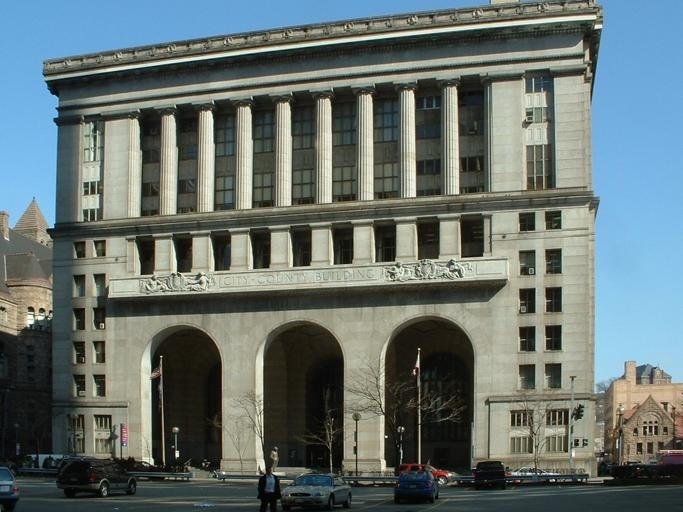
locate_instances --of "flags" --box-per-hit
[411,353,418,377]
[149,366,160,381]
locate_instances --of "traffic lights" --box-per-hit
[576,404,584,419]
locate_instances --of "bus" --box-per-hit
[21,453,63,470]
[657,449,682,478]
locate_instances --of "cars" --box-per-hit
[0,466,19,512]
[508,466,561,484]
[393,471,439,504]
[279,473,352,511]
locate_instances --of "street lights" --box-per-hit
[351,413,361,485]
[615,405,624,465]
[171,426,179,480]
[396,425,405,464]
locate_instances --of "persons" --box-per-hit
[270,446,279,472]
[145,275,168,294]
[449,259,464,278]
[387,262,404,280]
[188,272,210,291]
[256,466,281,512]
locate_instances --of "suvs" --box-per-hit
[623,460,641,465]
[394,463,455,485]
[55,460,137,498]
[475,460,506,489]
[56,456,93,475]
